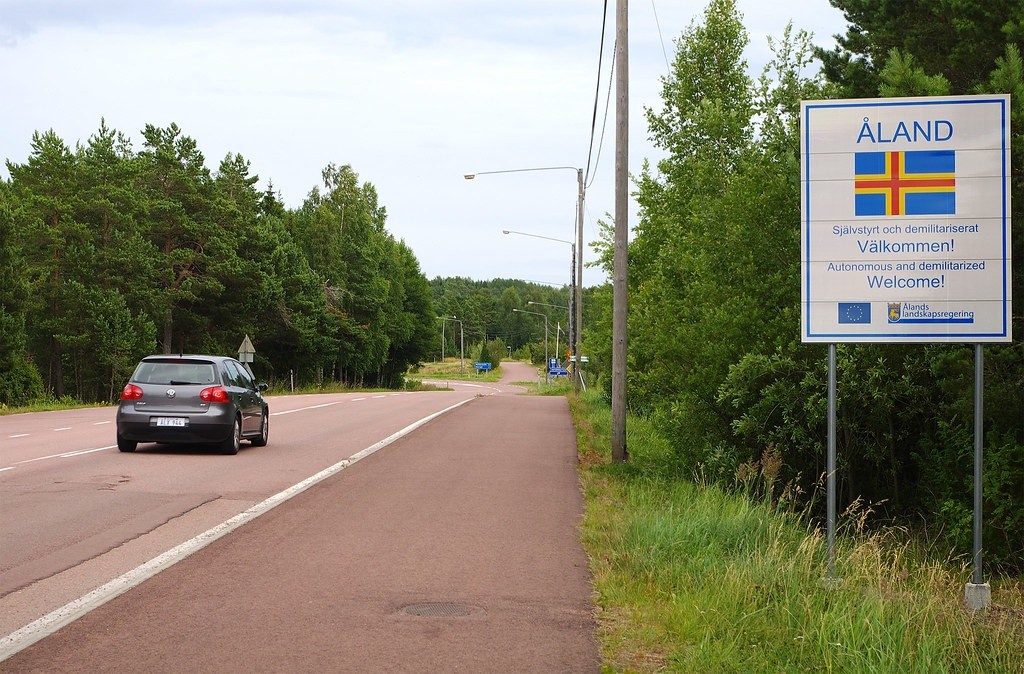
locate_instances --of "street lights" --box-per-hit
[502,230,575,384]
[529,301,572,359]
[513,309,548,385]
[464,167,581,397]
[435,317,463,378]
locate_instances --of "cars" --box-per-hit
[116,355,270,455]
